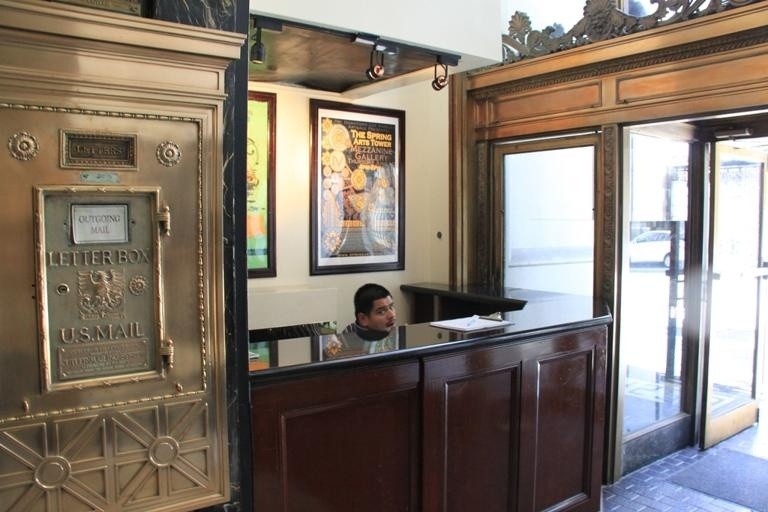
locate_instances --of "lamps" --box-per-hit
[250,28,267,65]
[432,64,450,90]
[366,47,385,81]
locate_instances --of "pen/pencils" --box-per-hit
[467,314,480,328]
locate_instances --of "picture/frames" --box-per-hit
[246,88,278,280]
[309,98,405,276]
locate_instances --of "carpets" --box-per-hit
[668,448,767,510]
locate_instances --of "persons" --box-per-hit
[340,284,396,334]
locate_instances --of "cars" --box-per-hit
[627,230,686,269]
